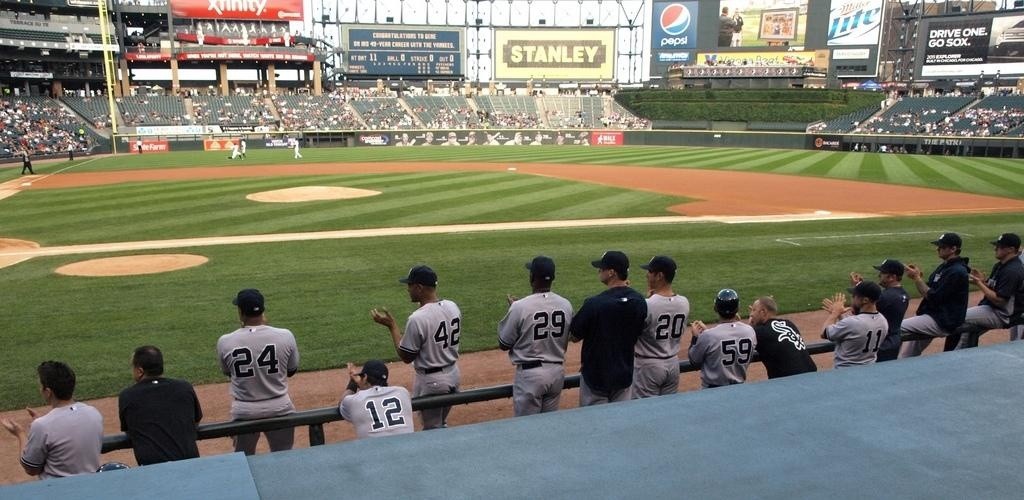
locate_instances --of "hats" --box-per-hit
[525,256,555,280]
[930,233,962,248]
[639,255,677,272]
[399,265,438,286]
[232,288,265,314]
[353,360,388,382]
[872,259,904,276]
[846,280,881,299]
[591,250,629,271]
[990,233,1021,247]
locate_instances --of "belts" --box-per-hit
[522,361,542,369]
[425,367,442,375]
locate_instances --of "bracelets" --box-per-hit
[347,378,357,392]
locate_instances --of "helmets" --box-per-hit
[715,288,739,314]
[96,461,130,473]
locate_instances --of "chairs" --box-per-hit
[817,94,1024,138]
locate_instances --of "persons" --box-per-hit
[225,143,243,160]
[962,233,1024,348]
[1,360,105,482]
[632,256,690,400]
[196,20,287,36]
[555,132,565,145]
[718,7,743,47]
[748,295,817,379]
[688,288,758,389]
[118,346,203,467]
[370,263,463,430]
[467,132,479,145]
[850,259,910,362]
[484,134,500,145]
[240,139,248,159]
[216,288,299,455]
[396,133,414,147]
[819,281,889,369]
[503,132,524,145]
[899,232,971,359]
[62,87,102,98]
[578,132,589,138]
[569,251,648,407]
[422,132,434,146]
[529,132,543,145]
[294,138,302,159]
[497,254,574,418]
[853,143,961,156]
[440,132,460,146]
[337,361,416,439]
[0,98,85,175]
[926,106,1024,138]
[137,138,142,152]
